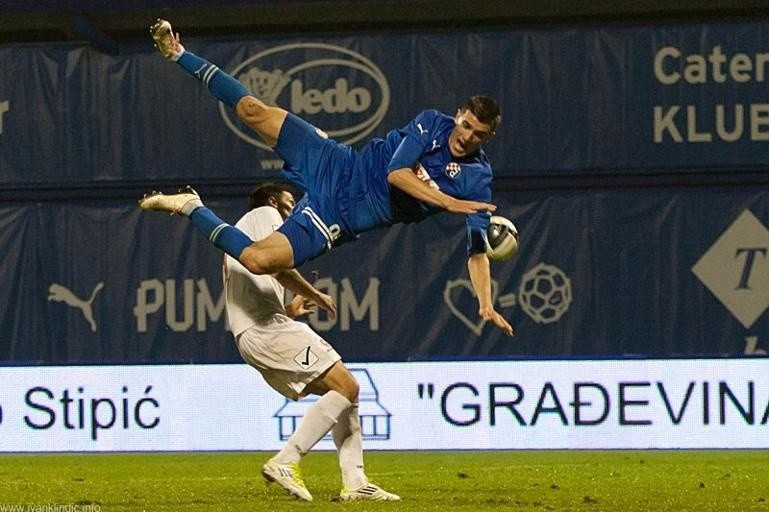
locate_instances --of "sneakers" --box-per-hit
[150,17,181,59]
[261,457,313,502]
[338,482,400,505]
[138,184,201,217]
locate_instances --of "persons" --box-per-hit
[137,17,514,339]
[220,180,403,503]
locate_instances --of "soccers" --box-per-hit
[489,216,518,260]
